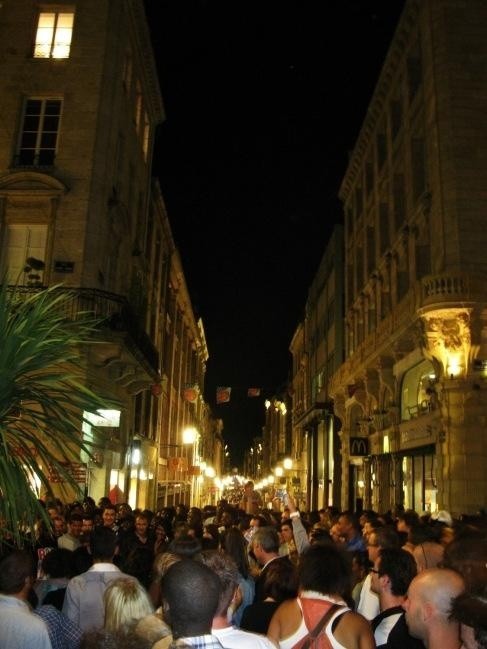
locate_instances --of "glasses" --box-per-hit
[362,541,375,547]
[368,566,378,575]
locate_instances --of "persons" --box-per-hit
[1,481,487,649]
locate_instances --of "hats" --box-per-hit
[430,509,451,525]
